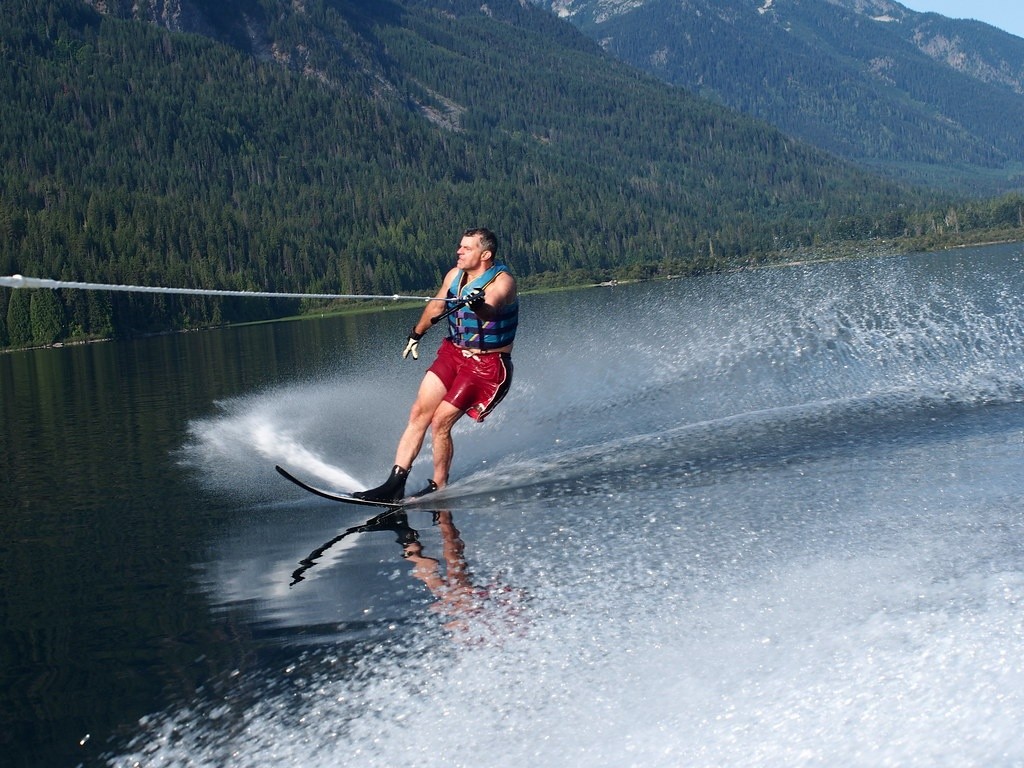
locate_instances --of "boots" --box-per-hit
[405,474,449,503]
[358,510,419,549]
[353,465,411,503]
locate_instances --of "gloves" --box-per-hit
[402,326,427,360]
[462,286,485,311]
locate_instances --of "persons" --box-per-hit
[353,227,518,503]
[364,510,536,648]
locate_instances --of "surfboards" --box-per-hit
[274,464,410,507]
